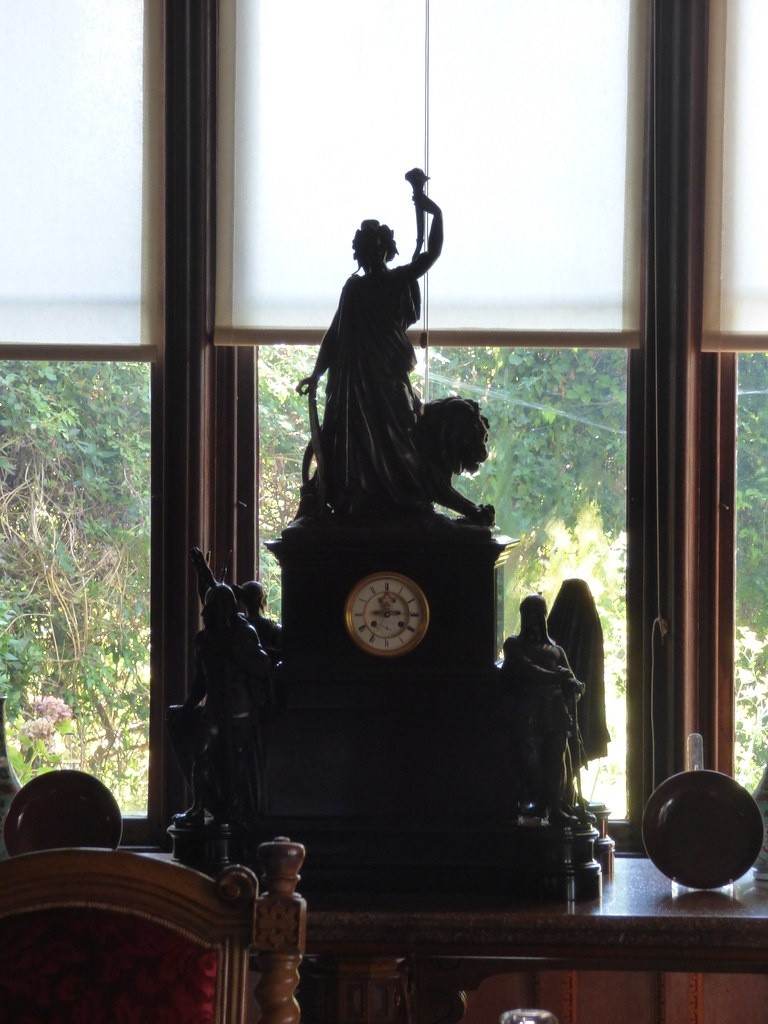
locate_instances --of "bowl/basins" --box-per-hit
[4,770,123,858]
[641,770,764,889]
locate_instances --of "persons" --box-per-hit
[294,193,443,490]
[171,584,277,826]
[190,548,292,666]
[503,596,586,815]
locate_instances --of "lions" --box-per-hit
[294,395,500,523]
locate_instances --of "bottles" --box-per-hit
[756,769,768,881]
[0,699,18,858]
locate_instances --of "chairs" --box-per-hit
[0,846,259,1024]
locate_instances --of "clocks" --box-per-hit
[345,570,430,657]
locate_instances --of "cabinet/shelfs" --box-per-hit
[296,940,768,1024]
